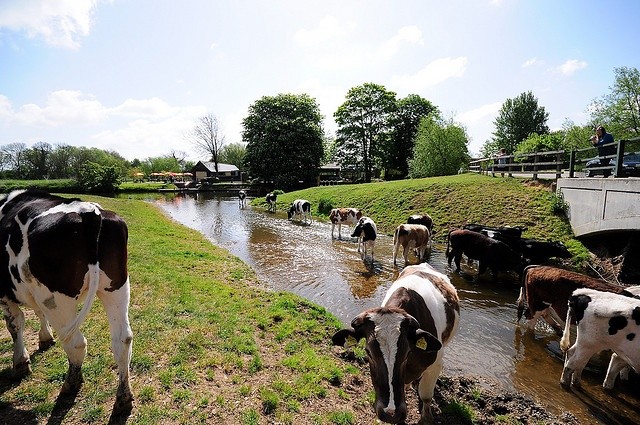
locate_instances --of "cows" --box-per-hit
[238,190,246,206]
[0,189,135,413]
[559,288,640,390]
[333,261,460,424]
[287,199,312,224]
[351,216,377,261]
[519,238,572,259]
[266,193,277,212]
[445,228,527,279]
[393,223,431,264]
[516,264,640,339]
[461,223,528,263]
[329,208,362,239]
[408,214,433,254]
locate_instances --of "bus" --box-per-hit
[317,166,341,183]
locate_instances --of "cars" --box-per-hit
[586,153,640,177]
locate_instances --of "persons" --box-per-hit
[591,125,615,178]
[498,148,510,178]
